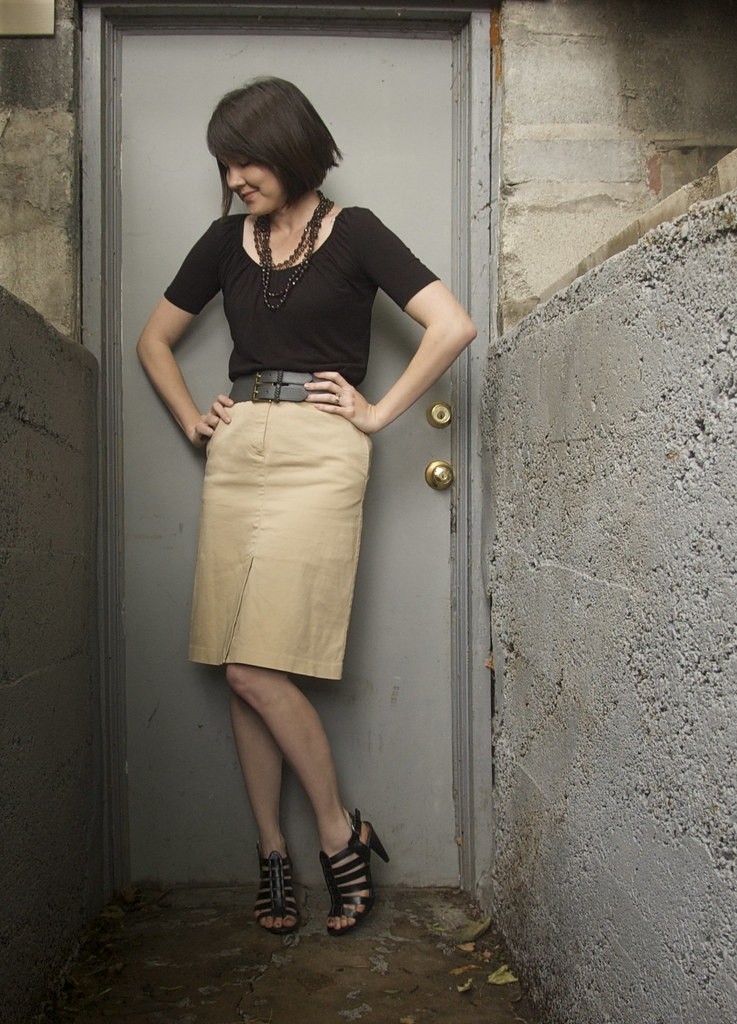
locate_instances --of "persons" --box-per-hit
[135,78,476,935]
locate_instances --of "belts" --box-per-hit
[229,370,335,403]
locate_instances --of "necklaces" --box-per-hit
[253,188,336,311]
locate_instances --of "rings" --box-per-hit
[334,393,340,406]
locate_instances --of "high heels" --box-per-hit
[253,839,300,935]
[319,808,390,937]
